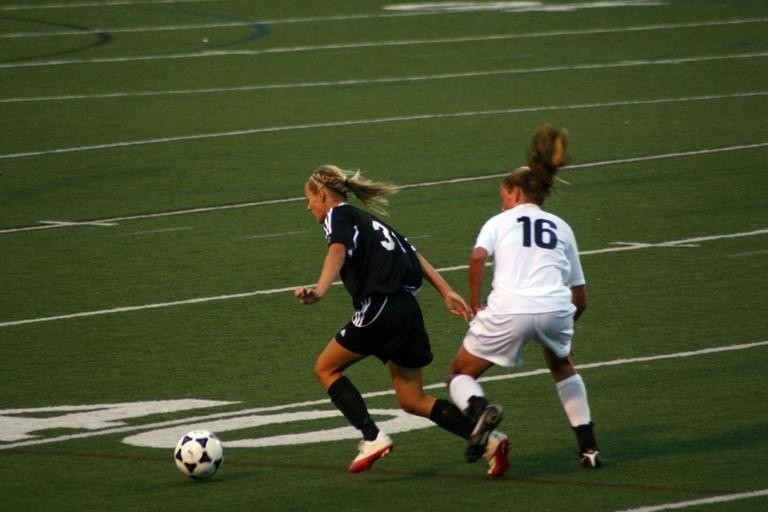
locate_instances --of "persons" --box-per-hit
[294,162,511,482]
[443,123,603,474]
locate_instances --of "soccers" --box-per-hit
[174,429,224,479]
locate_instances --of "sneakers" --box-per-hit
[580,449,603,470]
[483,429,511,480]
[348,429,394,474]
[464,395,503,464]
